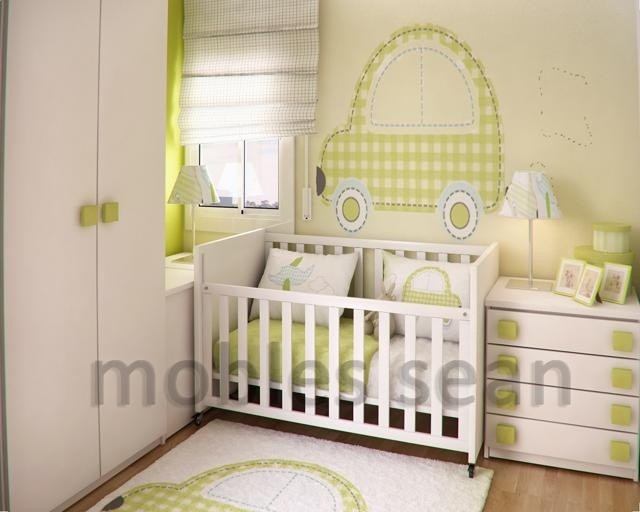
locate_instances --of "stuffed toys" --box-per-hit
[364,280,399,339]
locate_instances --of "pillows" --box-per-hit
[382,249,473,344]
[249,251,359,327]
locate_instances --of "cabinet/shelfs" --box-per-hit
[0,0,185,464]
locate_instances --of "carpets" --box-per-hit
[86,419,495,512]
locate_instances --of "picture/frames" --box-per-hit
[553,258,586,297]
[597,261,632,305]
[574,264,604,306]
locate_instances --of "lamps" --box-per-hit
[499,170,562,291]
[168,165,220,265]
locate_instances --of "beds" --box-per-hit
[194,221,498,478]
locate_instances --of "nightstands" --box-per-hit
[484,276,640,484]
